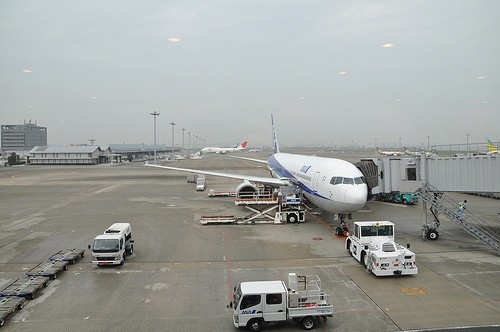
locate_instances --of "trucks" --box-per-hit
[230,272,333,332]
[187,174,206,191]
[88,222,135,266]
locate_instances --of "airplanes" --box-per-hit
[200,140,248,154]
[144,113,368,236]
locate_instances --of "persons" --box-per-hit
[342,225,348,238]
[456,200,468,221]
